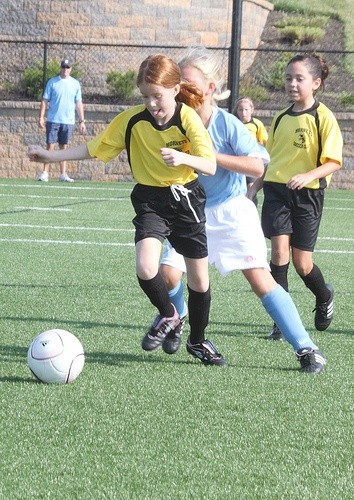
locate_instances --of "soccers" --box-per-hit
[27,329,85,383]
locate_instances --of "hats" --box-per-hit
[61,60,72,67]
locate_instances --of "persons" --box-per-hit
[230,96,268,207]
[25,54,225,366]
[37,58,86,182]
[246,51,344,341]
[158,47,328,376]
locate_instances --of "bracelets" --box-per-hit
[79,119,85,123]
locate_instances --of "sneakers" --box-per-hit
[142,302,180,351]
[38,172,48,181]
[312,284,334,330]
[59,174,73,182]
[186,336,225,367]
[162,318,184,354]
[295,347,326,373]
[270,324,285,342]
